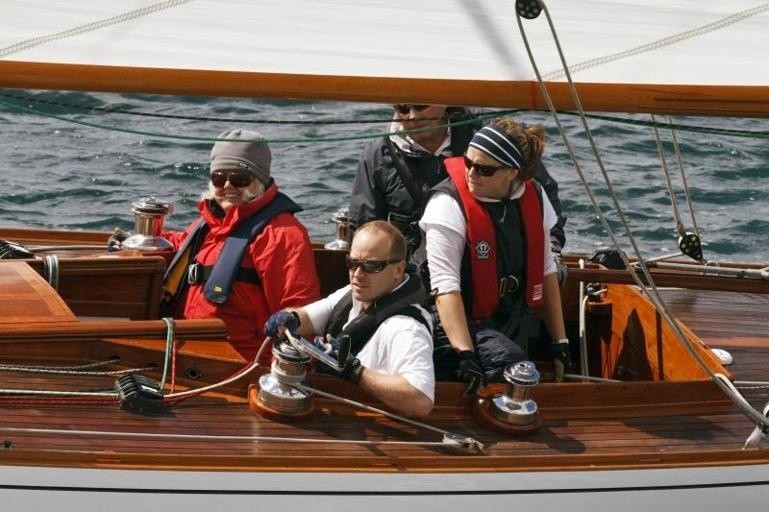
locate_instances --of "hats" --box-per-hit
[211,130,271,185]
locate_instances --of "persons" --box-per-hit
[335,103,569,288]
[107,128,322,374]
[262,220,436,419]
[418,116,572,400]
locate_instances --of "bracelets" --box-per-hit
[549,339,568,344]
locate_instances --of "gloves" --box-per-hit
[108,228,131,251]
[264,312,300,341]
[549,339,572,370]
[313,335,364,384]
[454,351,488,397]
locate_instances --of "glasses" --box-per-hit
[211,172,250,187]
[346,257,390,273]
[394,104,430,114]
[463,155,506,176]
